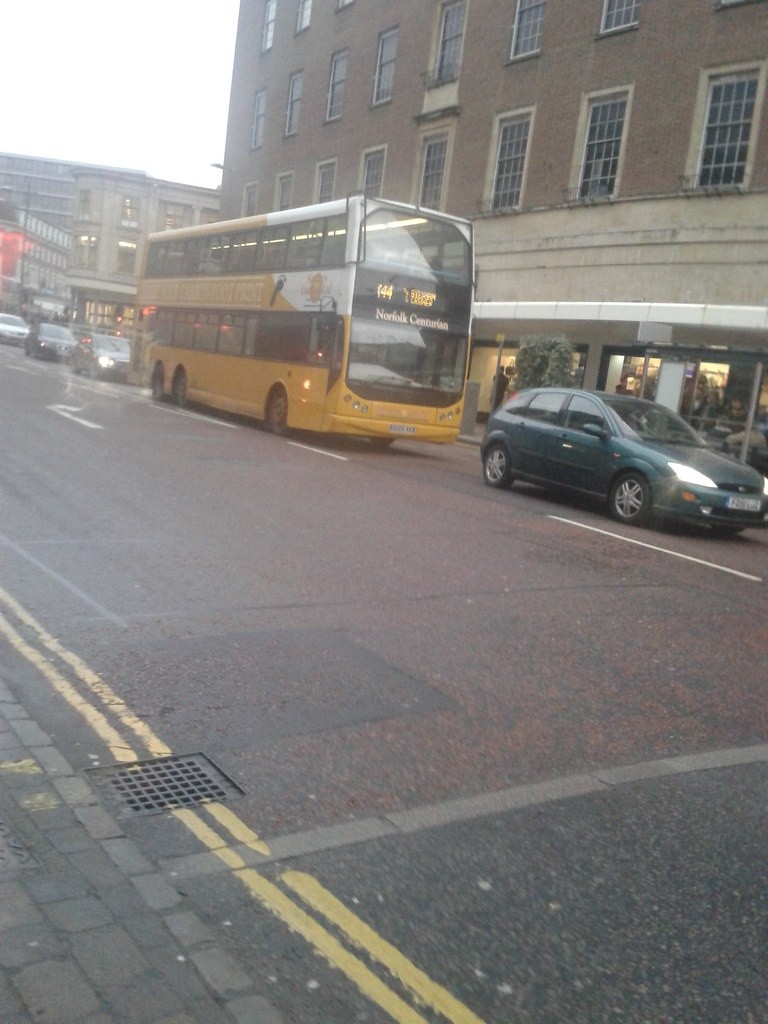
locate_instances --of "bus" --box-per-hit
[127,196,478,451]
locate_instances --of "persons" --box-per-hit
[616,385,655,400]
[490,366,509,410]
[350,342,376,364]
[41,311,70,322]
[685,382,768,475]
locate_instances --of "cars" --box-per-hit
[479,385,768,536]
[22,321,74,362]
[0,314,27,347]
[66,332,134,381]
